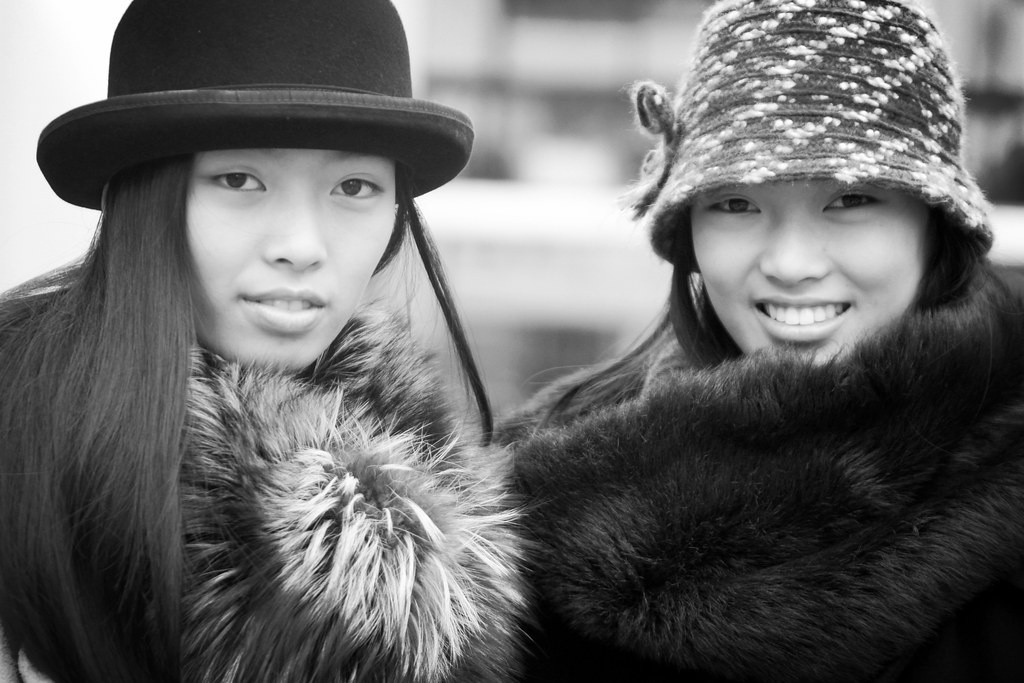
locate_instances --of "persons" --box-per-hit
[505,0,1024,683]
[0,0,535,683]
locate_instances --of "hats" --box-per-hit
[631,0,995,273]
[35,0,474,212]
